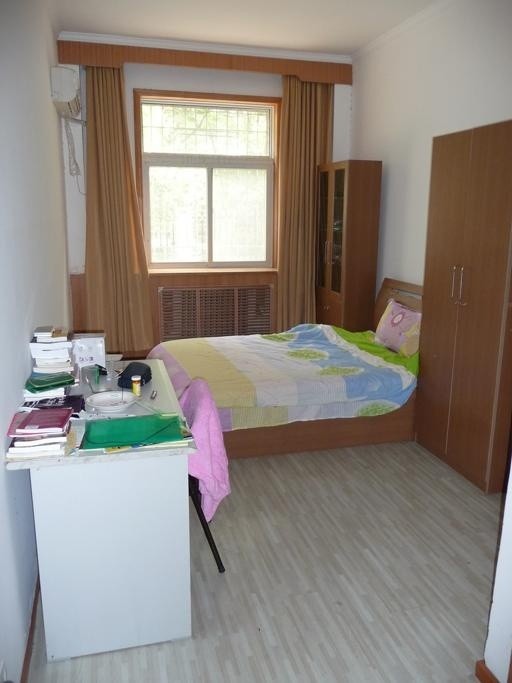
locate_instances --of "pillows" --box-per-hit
[375,300,422,353]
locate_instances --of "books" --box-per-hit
[6,327,79,461]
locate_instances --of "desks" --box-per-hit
[5,358,191,660]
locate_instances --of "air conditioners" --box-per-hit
[50,63,84,118]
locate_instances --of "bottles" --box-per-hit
[130,375,142,398]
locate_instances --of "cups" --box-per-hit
[81,366,100,399]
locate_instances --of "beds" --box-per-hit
[150,278,423,463]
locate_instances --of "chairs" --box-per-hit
[178,376,230,574]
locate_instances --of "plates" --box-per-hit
[87,391,138,412]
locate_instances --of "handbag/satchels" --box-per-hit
[117,361,152,388]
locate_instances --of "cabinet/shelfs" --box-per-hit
[316,159,381,332]
[413,118,512,495]
